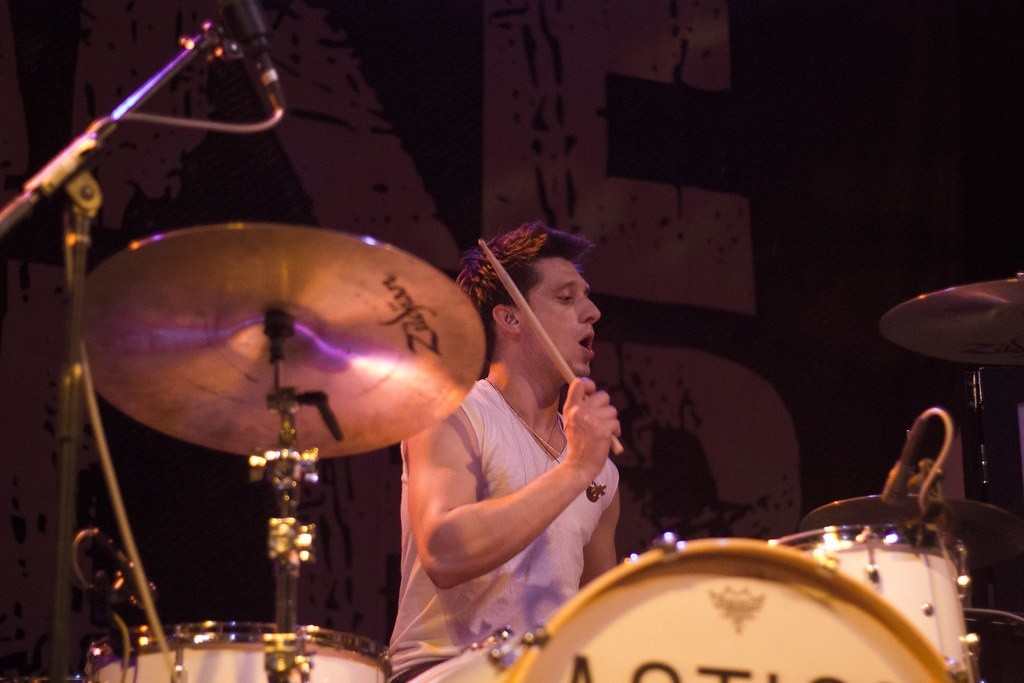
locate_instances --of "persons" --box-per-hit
[390,220,620,683]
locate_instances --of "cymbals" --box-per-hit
[79,220,489,461]
[876,267,1024,370]
[797,489,1024,679]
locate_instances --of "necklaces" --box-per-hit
[485,378,567,460]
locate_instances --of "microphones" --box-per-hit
[213,0,290,115]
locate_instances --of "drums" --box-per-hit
[74,612,400,683]
[766,524,984,683]
[403,625,532,681]
[499,526,961,683]
